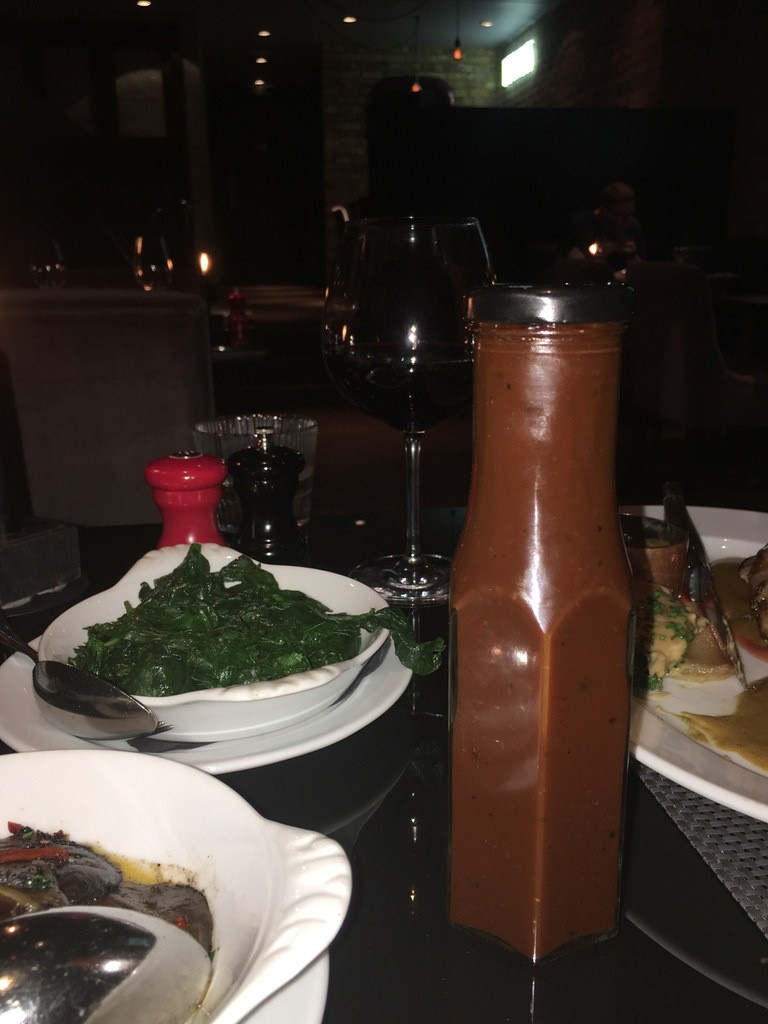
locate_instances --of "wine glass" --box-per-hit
[319,220,500,605]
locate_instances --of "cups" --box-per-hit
[193,415,317,534]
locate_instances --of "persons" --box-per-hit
[560,182,645,285]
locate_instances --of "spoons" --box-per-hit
[0,625,157,741]
[0,913,155,1024]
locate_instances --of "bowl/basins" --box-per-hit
[0,750,352,1024]
[620,515,689,593]
[37,546,392,741]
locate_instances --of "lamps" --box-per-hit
[410,15,422,93]
[453,0,464,61]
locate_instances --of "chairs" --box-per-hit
[626,259,768,506]
[1,286,228,530]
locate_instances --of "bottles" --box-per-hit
[447,282,630,961]
[144,449,227,548]
[228,427,307,568]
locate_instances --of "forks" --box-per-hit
[124,719,174,739]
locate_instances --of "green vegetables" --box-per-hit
[62,541,448,696]
[629,589,695,693]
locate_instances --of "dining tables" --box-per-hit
[0,511,768,1023]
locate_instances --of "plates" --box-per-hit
[616,504,768,824]
[0,636,413,771]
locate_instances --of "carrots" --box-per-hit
[0,821,67,863]
